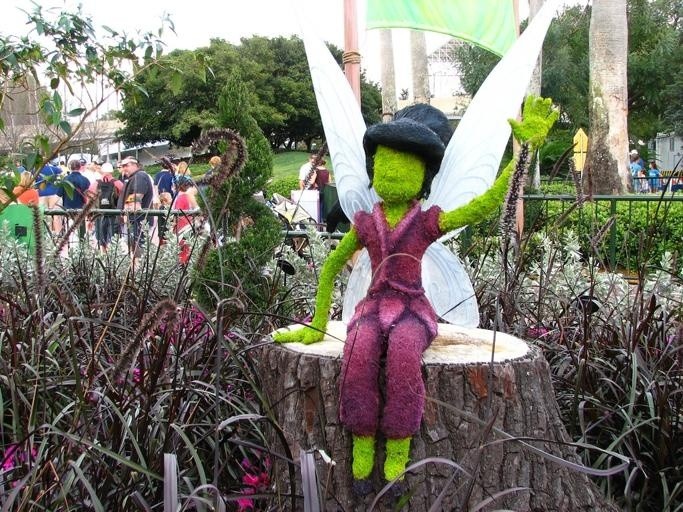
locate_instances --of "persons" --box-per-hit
[273,93,561,481]
[0,156,221,268]
[629,149,662,193]
[299,153,331,190]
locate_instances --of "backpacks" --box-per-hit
[97,179,117,209]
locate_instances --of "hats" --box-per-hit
[53,159,59,166]
[80,158,86,164]
[125,196,141,203]
[121,156,138,165]
[101,163,113,172]
